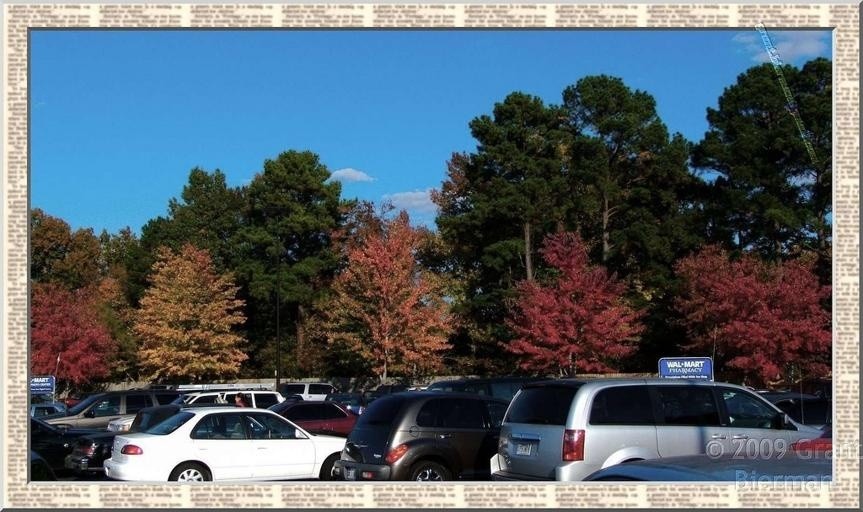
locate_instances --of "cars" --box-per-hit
[31,379,832,482]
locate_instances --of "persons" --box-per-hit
[235,392,249,408]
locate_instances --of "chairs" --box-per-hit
[194,422,275,440]
